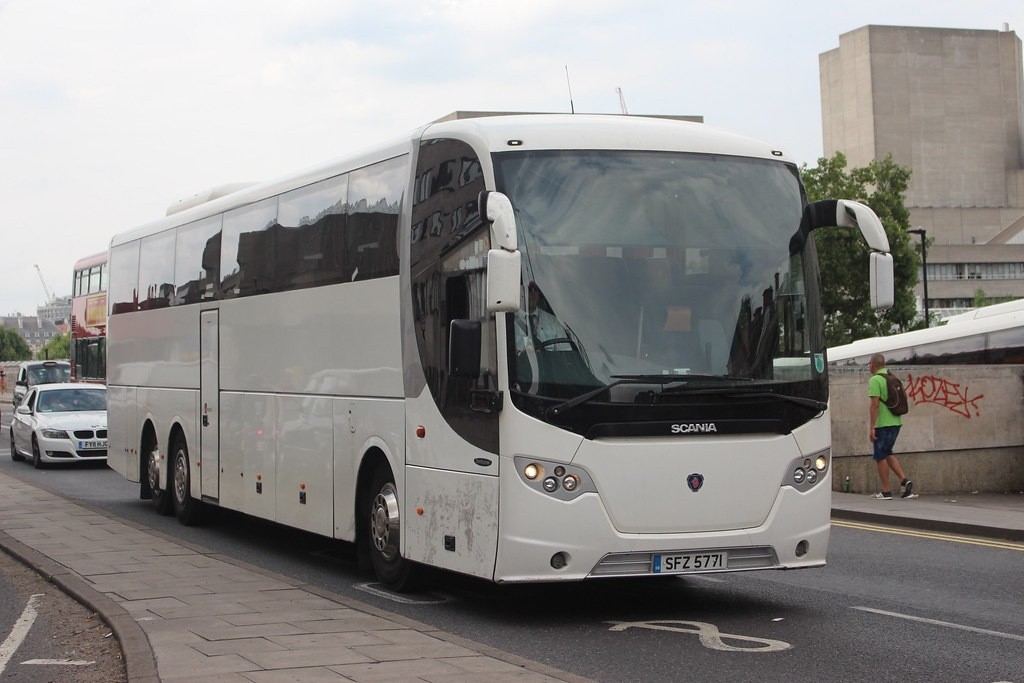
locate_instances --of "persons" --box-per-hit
[869,353,912,499]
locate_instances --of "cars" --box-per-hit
[9,384,107,468]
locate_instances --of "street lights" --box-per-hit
[907,229,930,328]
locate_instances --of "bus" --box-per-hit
[71,251,106,382]
[106,112,895,593]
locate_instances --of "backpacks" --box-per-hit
[875,370,909,416]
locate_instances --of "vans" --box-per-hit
[13,360,71,411]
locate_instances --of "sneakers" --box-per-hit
[900,478,913,498]
[870,492,892,499]
